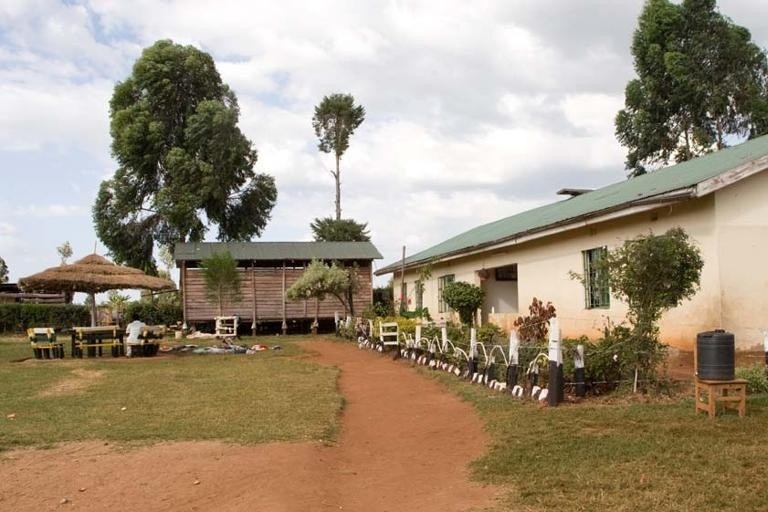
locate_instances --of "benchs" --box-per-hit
[27,325,166,358]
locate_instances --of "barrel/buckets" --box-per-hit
[175,331,182,340]
[696,328,735,382]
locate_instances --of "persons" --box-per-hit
[126,314,146,358]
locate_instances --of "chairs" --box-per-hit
[692,337,748,418]
